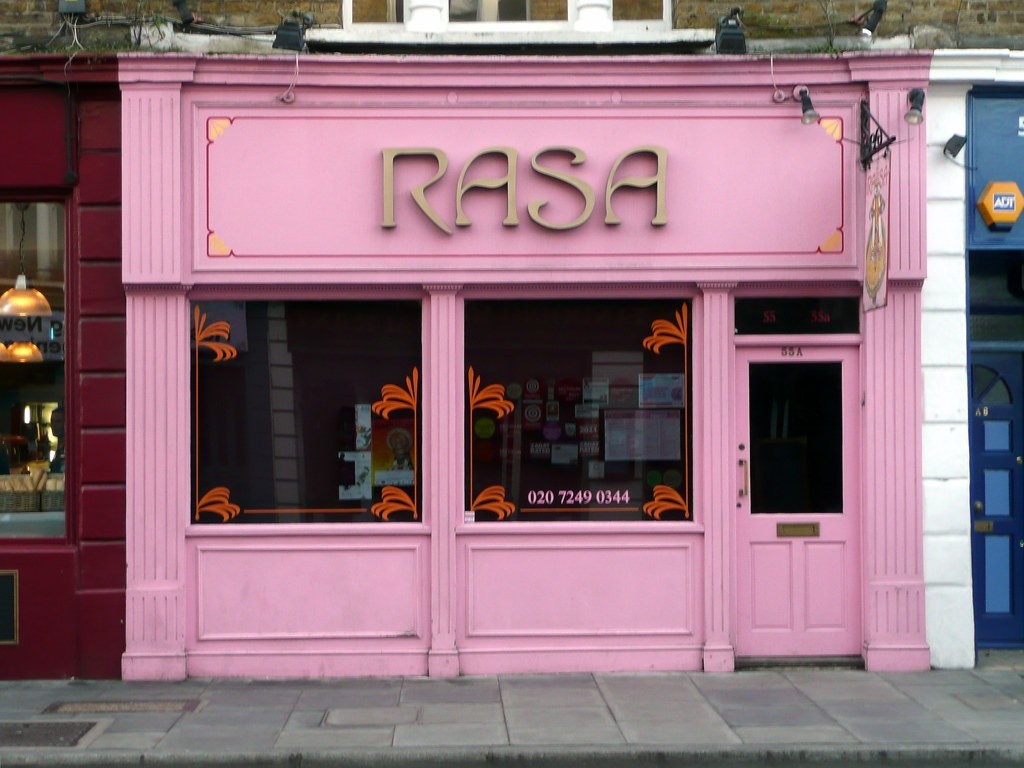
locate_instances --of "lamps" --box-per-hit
[0,202,54,317]
[856,0,888,36]
[7,342,44,363]
[945,134,967,156]
[800,88,820,124]
[271,9,315,51]
[903,88,926,125]
[714,7,748,55]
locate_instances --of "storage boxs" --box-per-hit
[0,474,42,512]
[41,473,67,512]
